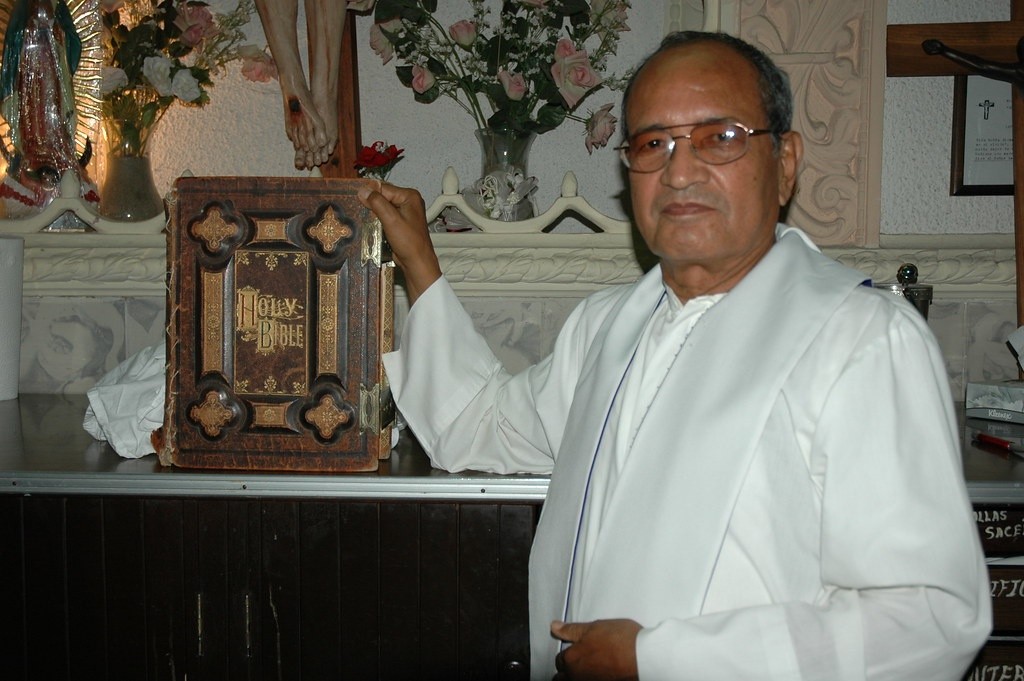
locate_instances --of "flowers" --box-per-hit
[347,0,639,162]
[357,142,405,180]
[99,0,279,156]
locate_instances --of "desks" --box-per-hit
[0,395,1024,681]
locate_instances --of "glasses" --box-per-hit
[613,120,771,174]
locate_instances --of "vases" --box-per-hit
[472,127,538,220]
[88,116,168,223]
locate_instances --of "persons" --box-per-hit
[0,0,101,228]
[254,0,349,168]
[354,31,994,681]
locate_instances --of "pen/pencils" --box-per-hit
[971,431,1024,454]
